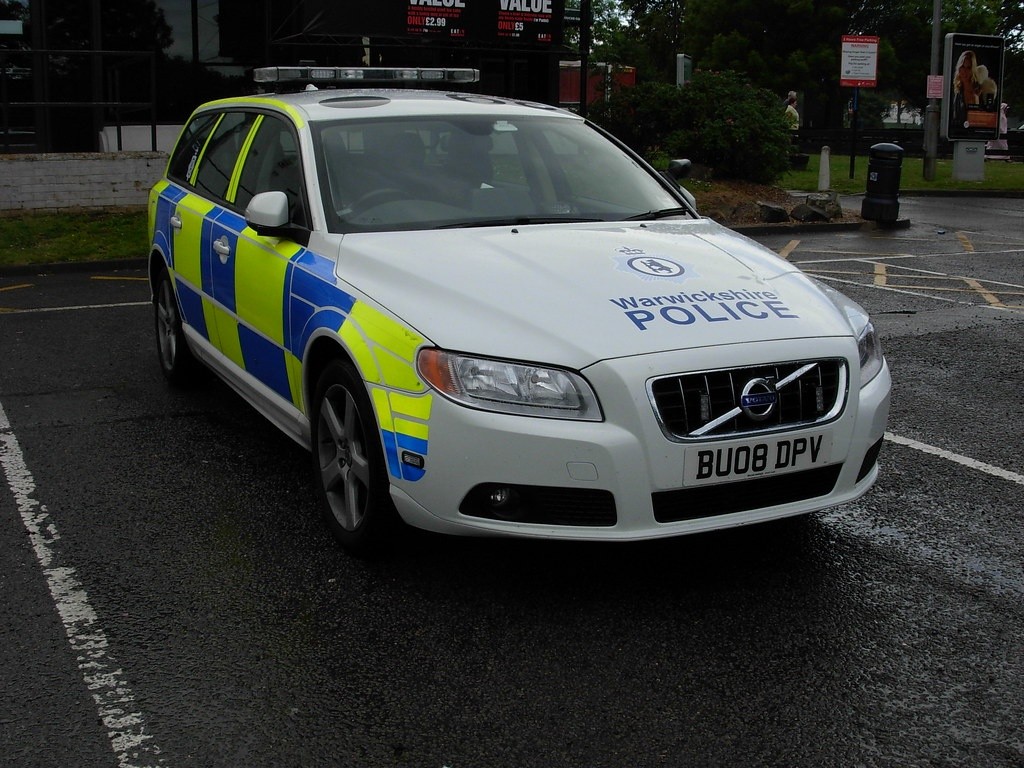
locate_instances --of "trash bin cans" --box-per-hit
[862,143,905,222]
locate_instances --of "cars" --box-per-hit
[146,66,892,555]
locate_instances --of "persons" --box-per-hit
[952,50,996,134]
[783,90,800,152]
[983,103,1015,164]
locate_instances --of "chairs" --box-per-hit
[386,134,427,170]
[272,166,303,206]
